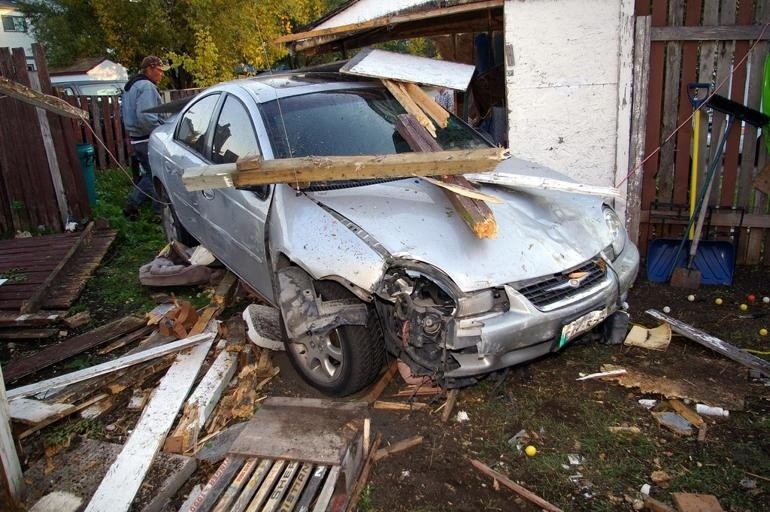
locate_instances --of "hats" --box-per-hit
[140,55,171,72]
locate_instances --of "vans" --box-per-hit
[52,81,129,109]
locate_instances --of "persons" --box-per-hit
[120,56,169,224]
[434,87,454,113]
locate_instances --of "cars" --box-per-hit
[148,72,640,397]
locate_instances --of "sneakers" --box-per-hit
[123,204,138,221]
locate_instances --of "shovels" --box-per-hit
[646,83,735,286]
[671,120,728,290]
